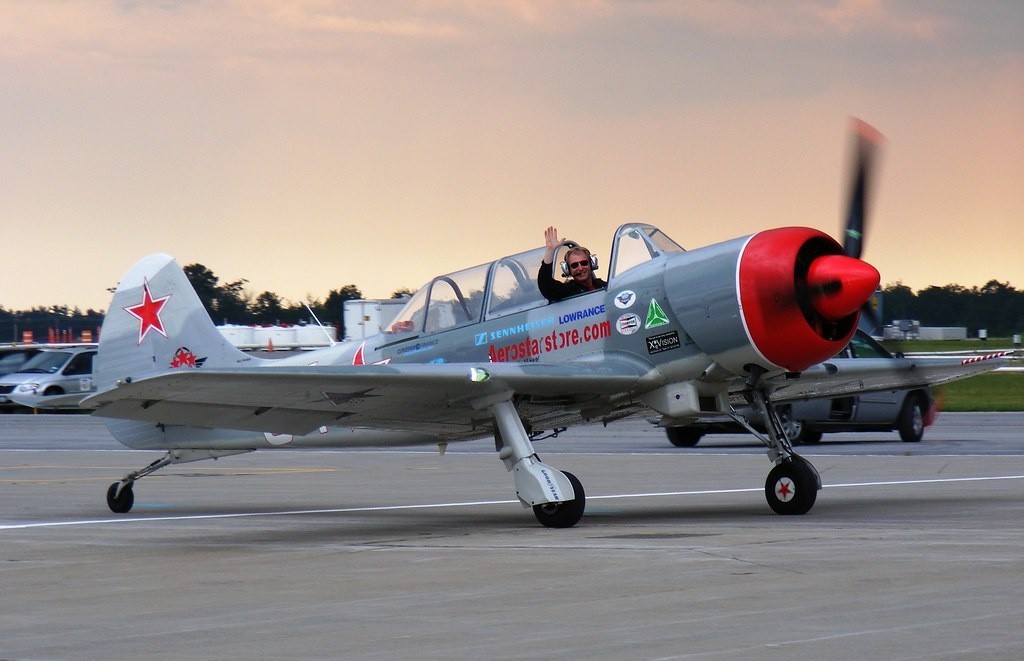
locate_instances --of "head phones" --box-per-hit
[560,246,599,277]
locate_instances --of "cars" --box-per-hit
[665,327,934,448]
[0,345,45,378]
[0,348,97,411]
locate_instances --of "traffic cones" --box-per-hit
[267,338,274,353]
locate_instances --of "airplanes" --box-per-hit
[79,115,1009,527]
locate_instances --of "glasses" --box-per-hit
[569,260,590,269]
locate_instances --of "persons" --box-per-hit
[538,226,610,306]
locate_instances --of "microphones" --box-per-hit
[572,273,581,277]
